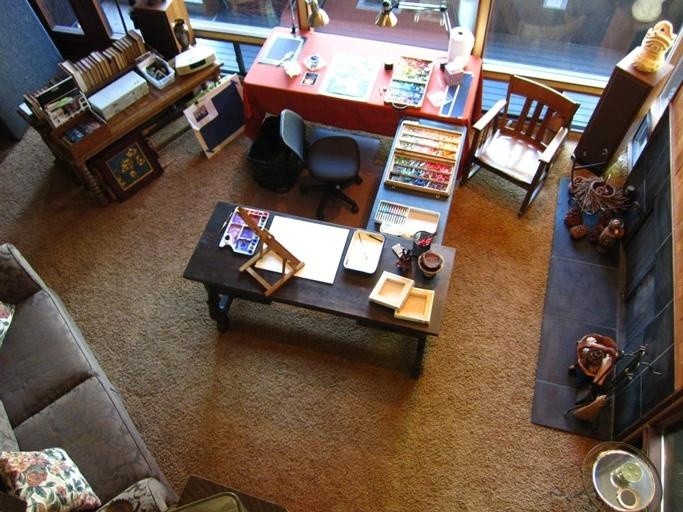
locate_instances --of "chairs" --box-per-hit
[278,107,362,219]
[464,66,580,217]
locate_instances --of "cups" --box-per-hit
[413,231,433,257]
[418,252,444,281]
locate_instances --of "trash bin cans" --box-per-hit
[246,116,304,194]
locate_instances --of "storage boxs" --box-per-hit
[88,70,151,120]
[138,52,176,91]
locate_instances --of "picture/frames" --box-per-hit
[83,129,166,202]
[394,286,436,323]
[369,270,415,313]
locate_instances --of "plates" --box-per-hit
[618,488,638,508]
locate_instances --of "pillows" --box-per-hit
[0,443,103,511]
[0,301,15,348]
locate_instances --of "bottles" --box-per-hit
[172,18,190,52]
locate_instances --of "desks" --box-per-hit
[41,51,226,206]
[575,442,663,511]
[242,25,484,183]
[365,108,470,246]
[183,201,457,382]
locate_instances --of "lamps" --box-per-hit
[288,1,330,35]
[377,0,454,47]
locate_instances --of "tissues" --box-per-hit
[443,56,467,87]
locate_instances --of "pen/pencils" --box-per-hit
[276,52,293,66]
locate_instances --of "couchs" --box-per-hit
[0,241,181,511]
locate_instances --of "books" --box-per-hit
[24,30,144,130]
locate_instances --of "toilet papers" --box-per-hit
[456,0,479,34]
[447,26,475,67]
